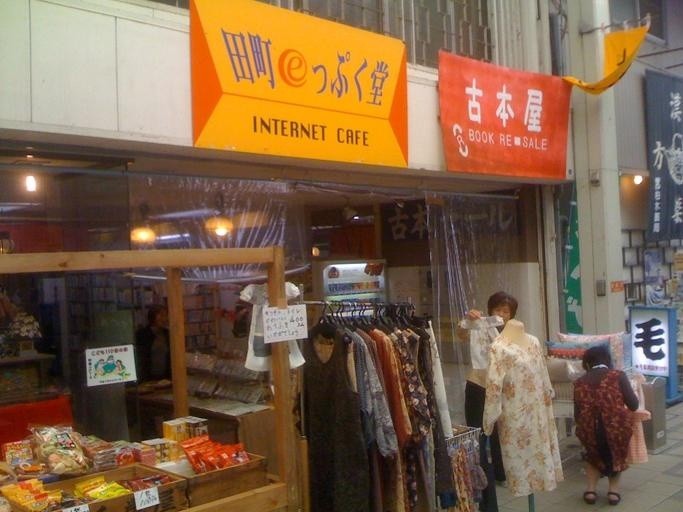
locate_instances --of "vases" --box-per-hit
[15,341,36,356]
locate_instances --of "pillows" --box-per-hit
[621,331,635,363]
[555,331,622,370]
[547,348,588,361]
[545,337,611,352]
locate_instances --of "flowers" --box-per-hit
[6,311,42,340]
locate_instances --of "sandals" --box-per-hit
[584,492,596,504]
[607,491,621,505]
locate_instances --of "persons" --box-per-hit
[456,291,519,486]
[136,304,172,384]
[573,346,640,505]
[482,319,565,497]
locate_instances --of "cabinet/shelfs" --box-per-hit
[1,437,287,511]
[309,257,390,314]
[74,283,222,356]
